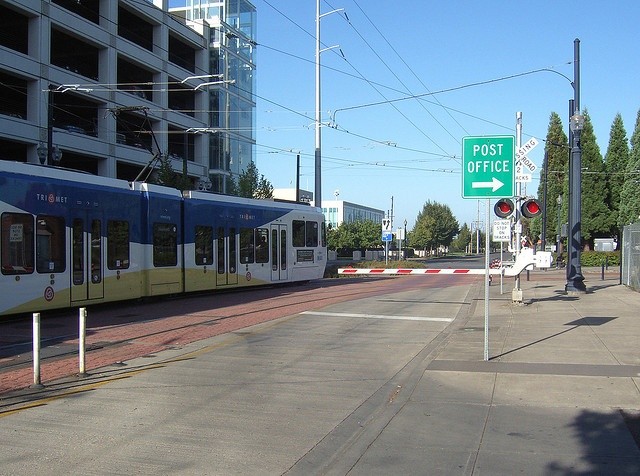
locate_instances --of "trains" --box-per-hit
[0,161,327,315]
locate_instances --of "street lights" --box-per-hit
[404,219,407,258]
[556,194,563,269]
[565,106,586,291]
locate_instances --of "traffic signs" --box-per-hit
[462,136,514,199]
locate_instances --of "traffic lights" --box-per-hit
[521,199,543,218]
[493,198,514,219]
